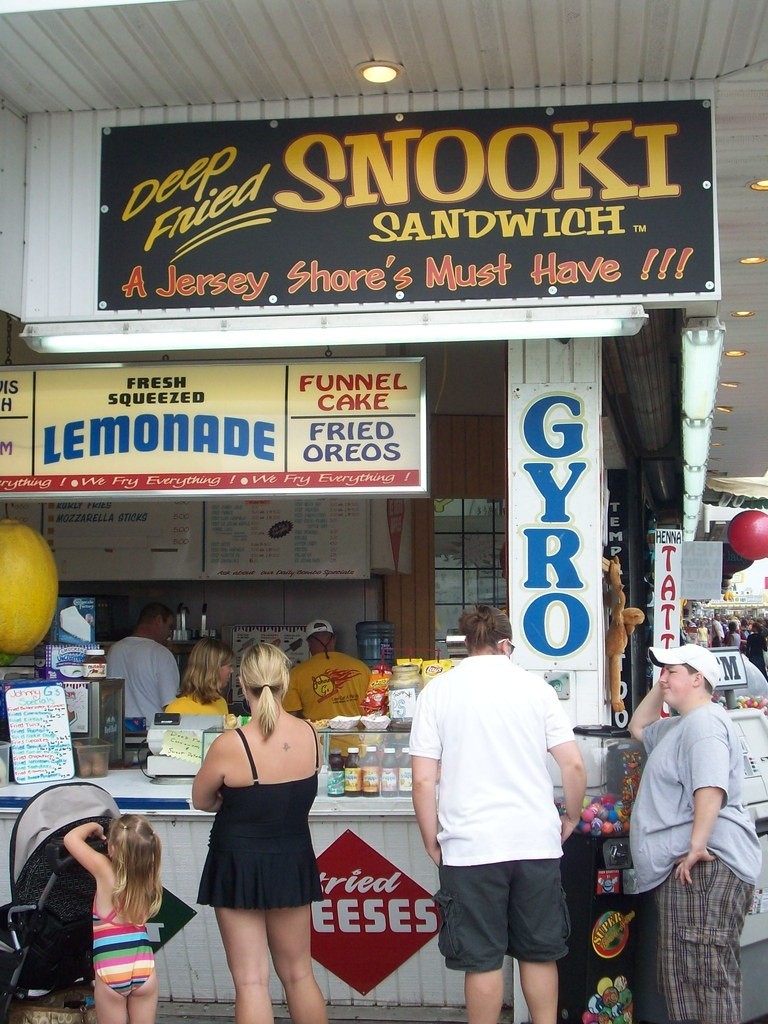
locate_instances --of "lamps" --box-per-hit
[17,304,647,354]
[680,314,725,542]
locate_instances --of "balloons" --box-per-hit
[728,510,768,561]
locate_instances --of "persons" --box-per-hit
[627,644,762,1024]
[409,606,587,1024]
[107,603,180,732]
[64,814,163,1024]
[192,644,327,1024]
[685,613,768,682]
[282,619,371,757]
[164,638,235,716]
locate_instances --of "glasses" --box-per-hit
[498,639,515,656]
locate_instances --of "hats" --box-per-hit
[648,643,721,692]
[305,620,334,640]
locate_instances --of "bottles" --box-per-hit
[388,664,424,732]
[328,749,345,797]
[345,747,362,797]
[362,746,381,797]
[82,649,107,681]
[381,748,399,797]
[399,747,413,796]
[356,621,395,668]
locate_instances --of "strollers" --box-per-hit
[0,782,125,1024]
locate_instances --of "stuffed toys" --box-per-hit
[604,556,645,714]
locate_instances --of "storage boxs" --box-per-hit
[71,736,114,778]
[0,740,11,788]
[33,645,101,680]
